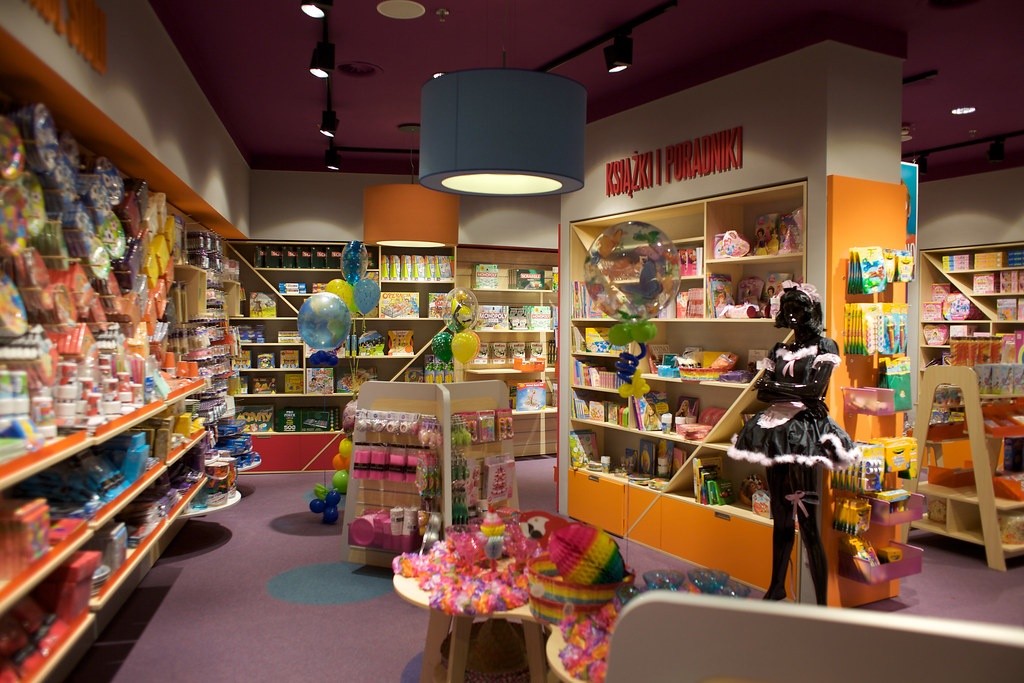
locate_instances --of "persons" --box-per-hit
[725,279,864,605]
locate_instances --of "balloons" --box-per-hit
[582,220,681,399]
[308,437,352,525]
[429,287,482,366]
[295,239,382,366]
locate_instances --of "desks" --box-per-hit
[391,566,611,683]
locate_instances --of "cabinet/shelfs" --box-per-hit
[456,250,559,462]
[1,378,207,683]
[236,240,455,471]
[919,243,1024,367]
[571,181,804,602]
[917,367,1023,571]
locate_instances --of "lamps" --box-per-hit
[987,139,1007,161]
[604,35,633,73]
[316,97,341,140]
[309,32,334,80]
[326,149,343,172]
[417,67,585,200]
[915,156,929,173]
[300,0,331,18]
